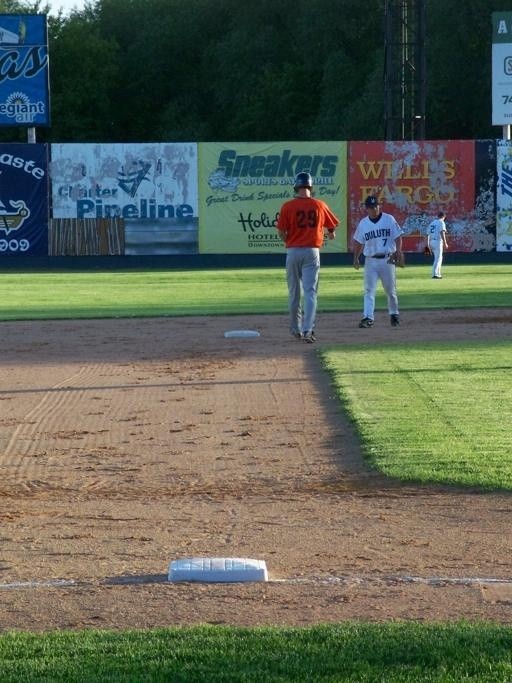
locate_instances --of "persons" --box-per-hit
[351,195,405,331]
[425,211,450,280]
[275,171,341,343]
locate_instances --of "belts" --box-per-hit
[371,253,390,259]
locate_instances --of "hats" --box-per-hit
[365,196,380,208]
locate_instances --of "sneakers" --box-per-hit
[390,314,400,327]
[289,326,302,339]
[432,274,442,278]
[358,318,375,328]
[302,331,317,343]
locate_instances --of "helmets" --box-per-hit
[294,171,313,190]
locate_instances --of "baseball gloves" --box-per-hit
[424,246,432,255]
[387,251,404,267]
